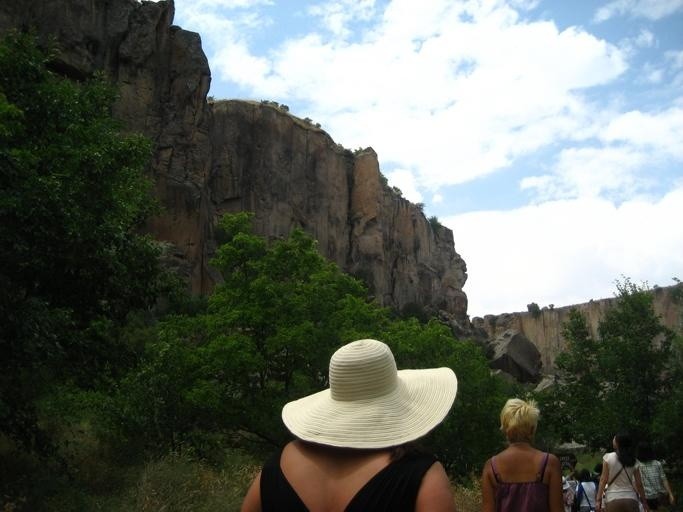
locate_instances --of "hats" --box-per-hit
[594,463,603,474]
[561,476,570,490]
[281,339,458,450]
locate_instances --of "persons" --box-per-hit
[635,440,677,511]
[237,437,458,512]
[592,431,651,511]
[477,396,567,511]
[561,458,607,511]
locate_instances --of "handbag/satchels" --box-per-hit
[577,484,584,504]
[655,494,679,511]
[564,488,574,506]
[589,508,596,512]
